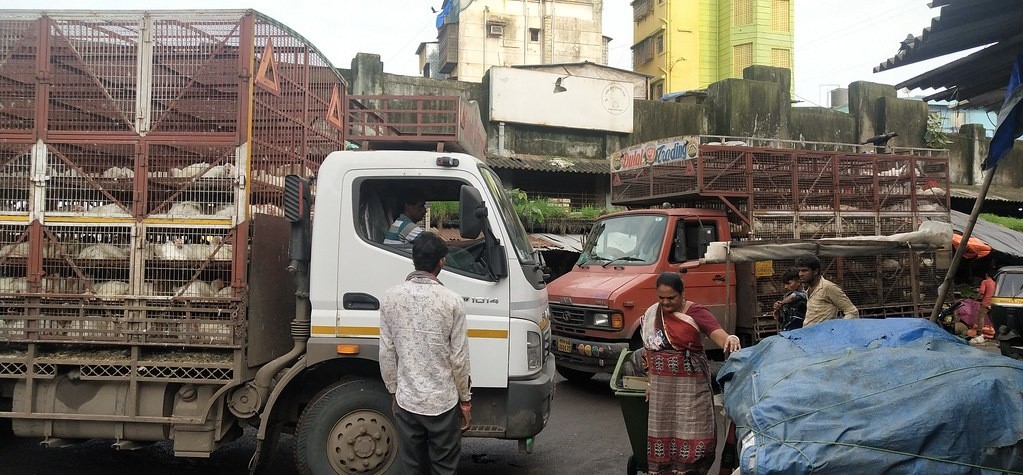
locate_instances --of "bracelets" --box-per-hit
[648,383,650,386]
[459,403,471,411]
[727,335,739,341]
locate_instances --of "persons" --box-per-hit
[379,231,472,475]
[970,271,995,343]
[383,191,485,244]
[773,253,859,331]
[639,272,742,475]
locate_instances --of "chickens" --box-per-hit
[758,246,935,310]
[723,158,946,234]
[1,159,314,345]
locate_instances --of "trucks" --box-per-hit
[548,133,956,383]
[0,9,560,475]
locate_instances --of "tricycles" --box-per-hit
[986,265,1023,358]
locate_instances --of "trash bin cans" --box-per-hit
[610,348,649,475]
